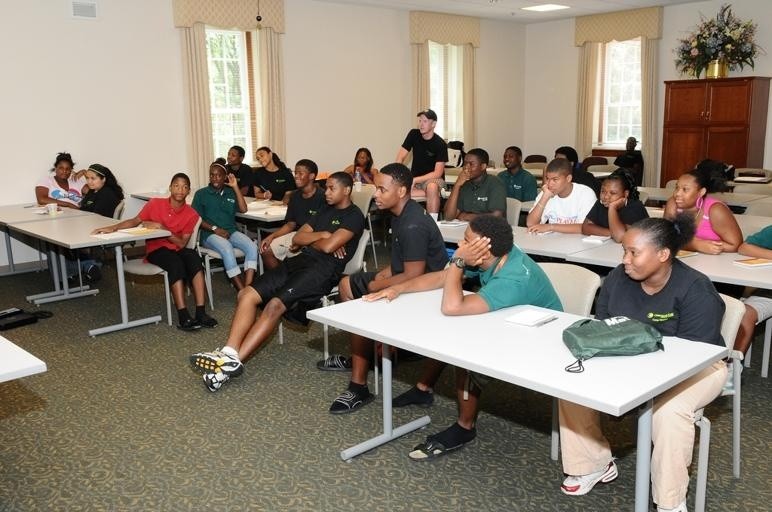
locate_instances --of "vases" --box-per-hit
[706,58,729,77]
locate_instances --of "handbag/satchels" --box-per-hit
[561,313,665,365]
[445,146,463,168]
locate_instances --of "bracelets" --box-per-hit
[537,202,544,208]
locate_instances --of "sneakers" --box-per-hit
[198,313,219,329]
[720,363,743,397]
[204,372,230,392]
[176,317,200,331]
[190,352,244,376]
[81,262,102,282]
[555,461,619,498]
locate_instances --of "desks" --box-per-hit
[0,201,171,336]
[0,333,47,383]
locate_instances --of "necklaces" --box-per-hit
[694,208,701,220]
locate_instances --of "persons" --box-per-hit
[344,147,385,248]
[558,211,728,512]
[396,109,448,223]
[317,161,450,414]
[718,224,772,395]
[225,146,296,240]
[553,147,599,197]
[36,152,125,291]
[443,149,506,222]
[88,173,218,331]
[526,158,597,233]
[191,158,258,300]
[500,147,536,228]
[362,216,564,461]
[189,171,366,394]
[664,168,743,254]
[614,138,643,182]
[260,159,326,310]
[581,168,648,243]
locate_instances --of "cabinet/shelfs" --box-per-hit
[660,78,753,187]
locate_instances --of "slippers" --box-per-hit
[314,353,353,372]
[326,387,376,415]
[408,433,464,460]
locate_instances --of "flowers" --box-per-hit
[677,13,760,71]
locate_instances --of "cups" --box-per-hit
[46,203,57,217]
[354,182,362,192]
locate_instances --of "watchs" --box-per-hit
[449,258,467,269]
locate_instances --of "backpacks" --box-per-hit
[447,141,466,168]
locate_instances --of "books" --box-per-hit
[580,233,614,244]
[733,258,772,267]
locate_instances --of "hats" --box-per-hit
[626,136,639,143]
[416,109,439,120]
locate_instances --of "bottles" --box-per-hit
[355,167,361,181]
